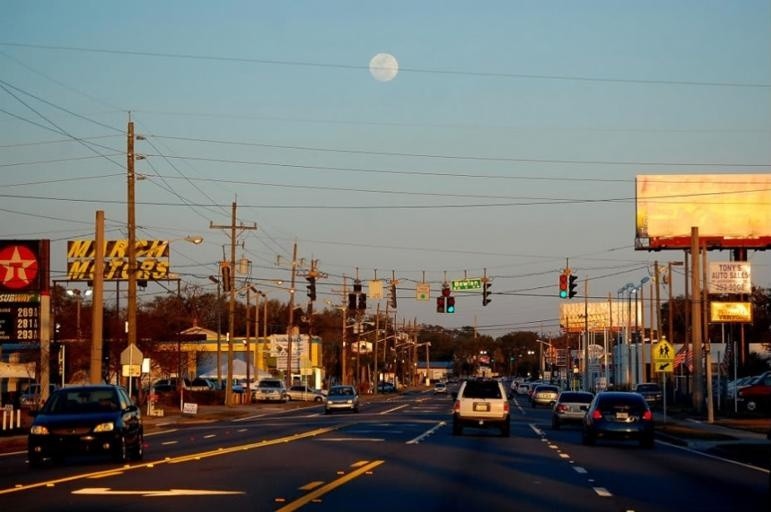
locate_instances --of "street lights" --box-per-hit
[254,287,298,381]
[67,288,93,336]
[125,235,204,385]
[616,259,686,416]
[225,279,282,406]
[536,339,554,383]
[286,297,332,384]
[341,320,433,395]
[307,306,348,387]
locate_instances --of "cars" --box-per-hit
[17,383,58,410]
[726,370,771,412]
[154,377,216,391]
[325,385,360,413]
[501,376,663,448]
[433,383,447,395]
[255,378,284,402]
[27,382,144,466]
[282,386,327,403]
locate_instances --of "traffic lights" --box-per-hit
[348,293,357,309]
[448,297,456,313]
[569,274,579,299]
[354,285,361,291]
[437,297,445,312]
[443,288,450,296]
[307,277,315,301]
[359,294,366,309]
[483,281,492,305]
[387,284,397,309]
[560,275,568,299]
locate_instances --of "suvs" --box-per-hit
[450,380,515,436]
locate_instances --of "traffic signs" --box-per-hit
[452,278,481,292]
